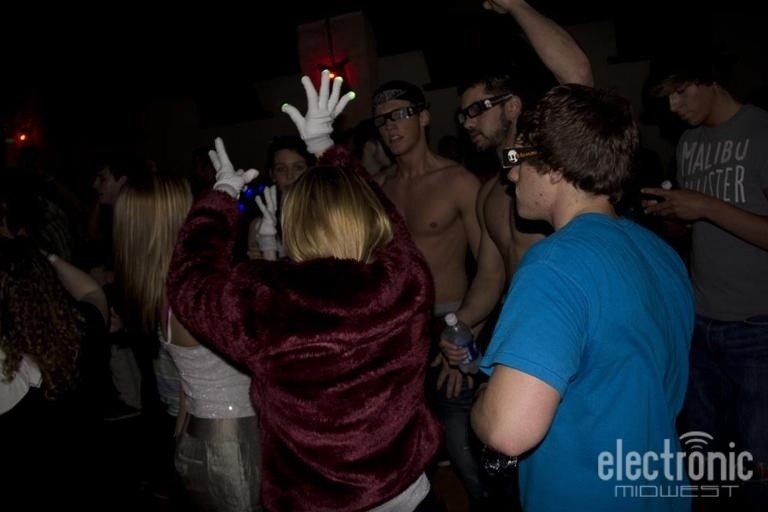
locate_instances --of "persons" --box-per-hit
[468,81,698,512]
[439,0,598,372]
[0,66,488,512]
[638,45,767,481]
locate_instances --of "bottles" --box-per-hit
[443,312,483,375]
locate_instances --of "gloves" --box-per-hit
[281,70,355,152]
[256,187,276,253]
[208,138,259,200]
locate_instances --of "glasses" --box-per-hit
[499,145,539,169]
[374,104,419,127]
[457,93,511,123]
